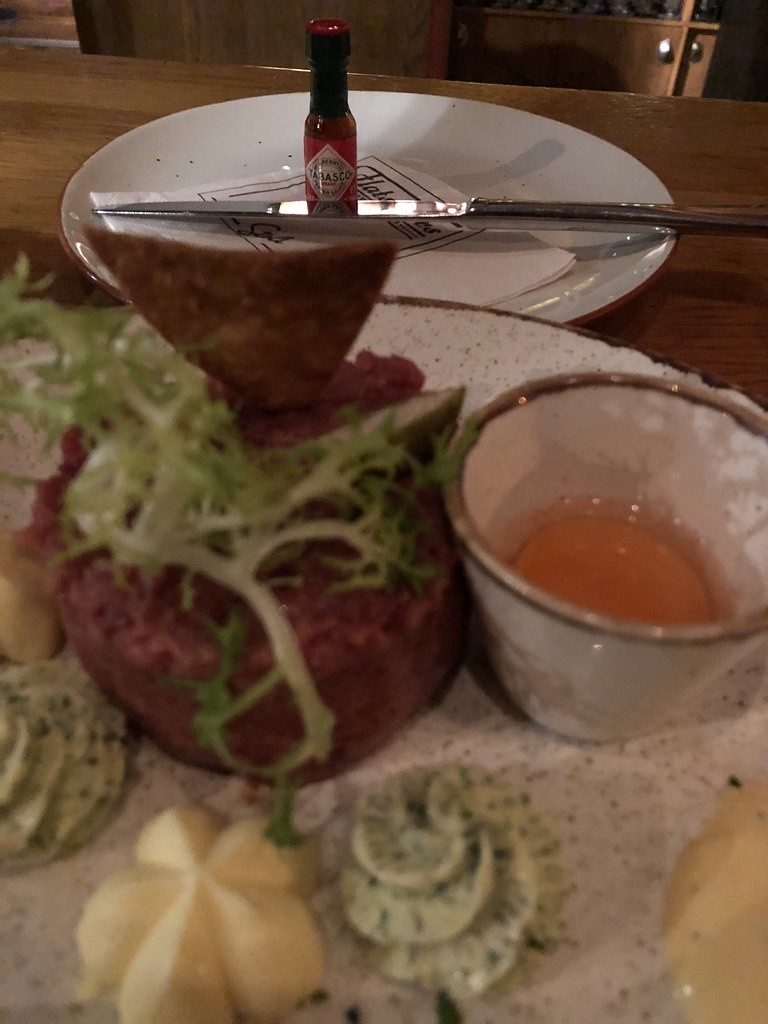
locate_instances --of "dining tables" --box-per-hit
[1,45,768,1022]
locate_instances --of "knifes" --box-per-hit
[91,202,768,236]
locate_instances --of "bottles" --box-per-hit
[305,17,358,221]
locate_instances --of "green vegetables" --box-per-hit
[0,253,484,854]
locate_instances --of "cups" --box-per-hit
[445,367,768,743]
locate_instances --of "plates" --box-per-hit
[60,88,678,330]
[0,295,768,1024]
